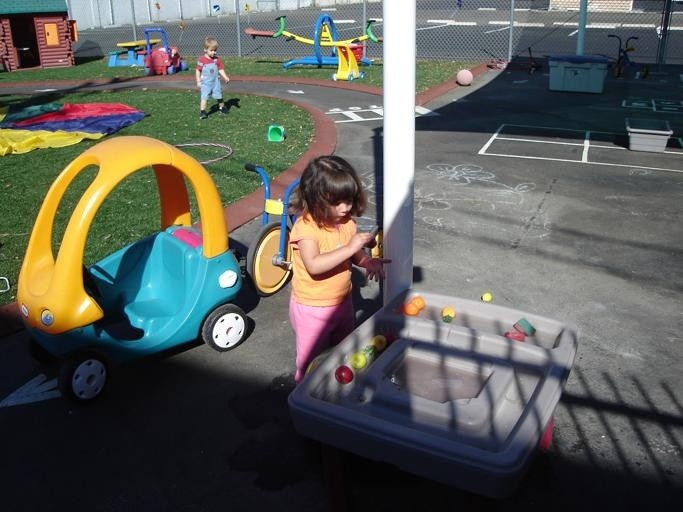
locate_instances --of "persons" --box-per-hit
[195,38,230,119]
[288,155,393,386]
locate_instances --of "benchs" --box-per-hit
[108,49,157,71]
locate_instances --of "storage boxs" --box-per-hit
[546,51,608,95]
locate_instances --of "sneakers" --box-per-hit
[200,110,208,118]
[218,107,228,114]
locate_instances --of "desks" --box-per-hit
[116,37,163,70]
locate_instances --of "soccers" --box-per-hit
[457,69,473,85]
